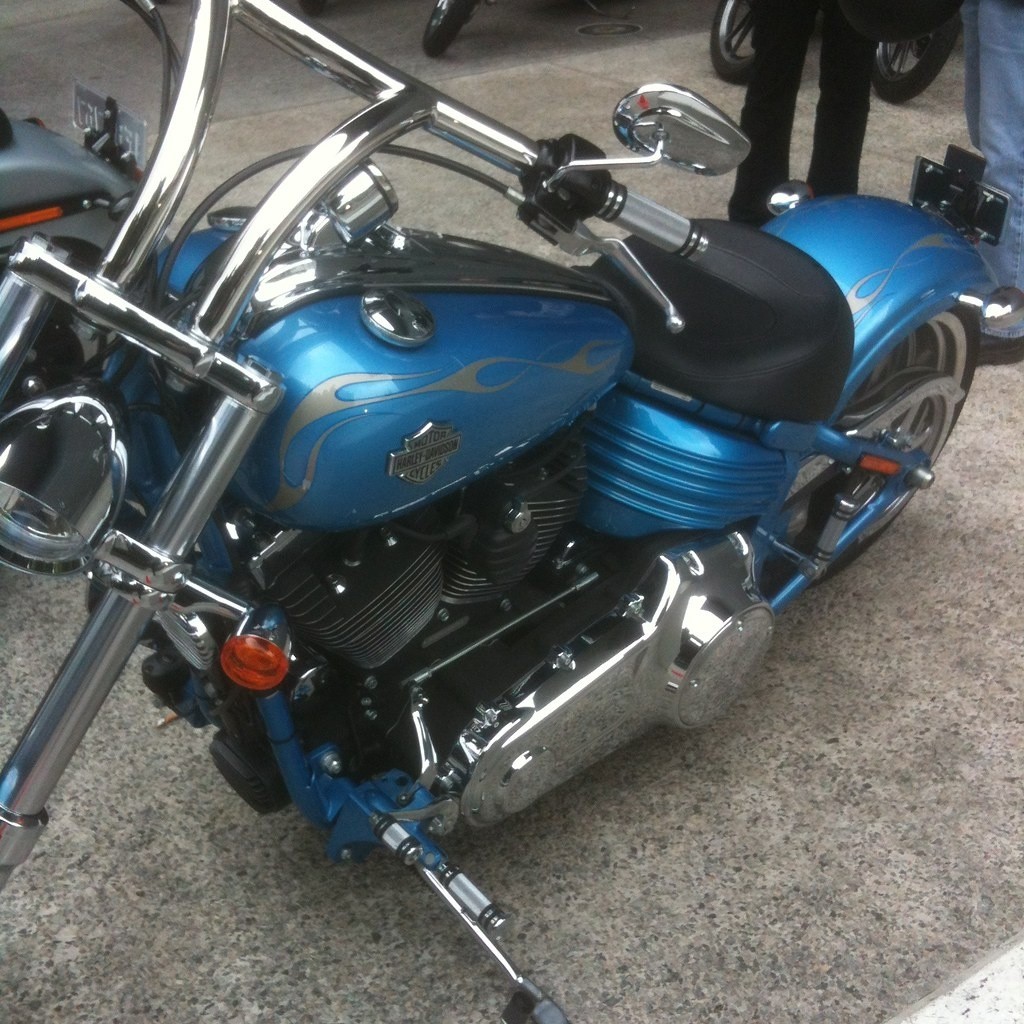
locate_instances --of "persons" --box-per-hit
[726,0,884,225]
[959,1,1024,367]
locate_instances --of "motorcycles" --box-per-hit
[2,5,1024,1024]
